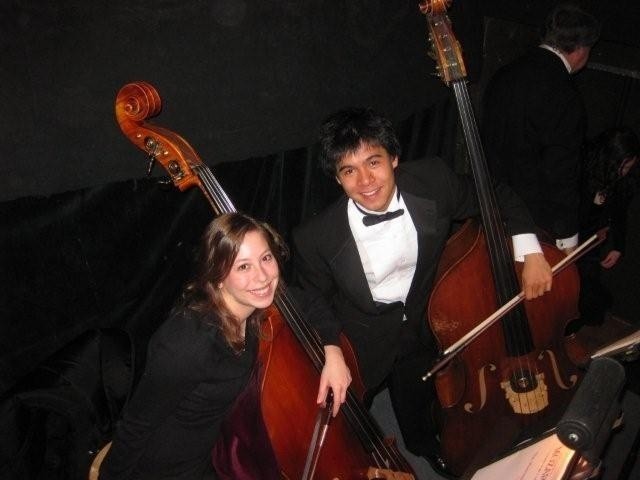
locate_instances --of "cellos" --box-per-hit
[115,81,418,480]
[417,0,589,480]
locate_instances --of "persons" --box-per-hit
[107,213,354,480]
[581,127,640,319]
[292,109,552,463]
[487,6,595,327]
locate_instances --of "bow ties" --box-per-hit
[363,209,404,227]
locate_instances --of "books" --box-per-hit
[590,329,640,360]
[469,428,580,480]
